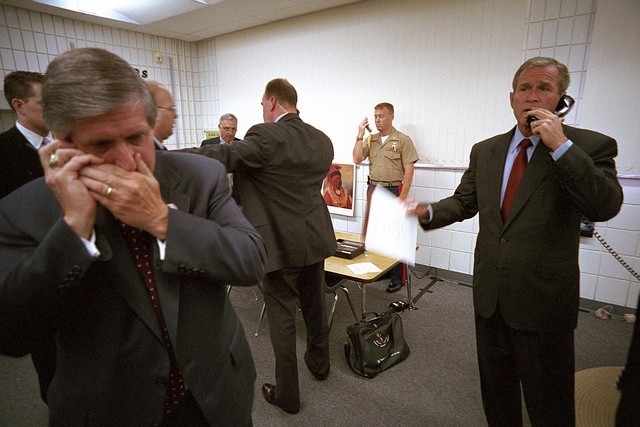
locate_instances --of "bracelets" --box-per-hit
[357,135,363,142]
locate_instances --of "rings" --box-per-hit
[105,187,112,197]
[47,154,60,164]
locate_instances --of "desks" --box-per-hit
[324,229,419,322]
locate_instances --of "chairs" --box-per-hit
[254,276,359,339]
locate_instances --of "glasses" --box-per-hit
[157,104,178,114]
[220,122,237,132]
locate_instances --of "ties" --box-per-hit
[42,137,51,145]
[118,219,186,427]
[501,138,534,221]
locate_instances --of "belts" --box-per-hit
[368,179,401,186]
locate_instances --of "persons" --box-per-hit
[140,80,176,150]
[416,55,624,425]
[1,70,54,202]
[353,103,419,292]
[169,79,336,414]
[1,48,268,426]
[199,113,242,146]
[322,167,351,207]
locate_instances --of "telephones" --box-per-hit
[527,94,576,131]
[333,238,365,259]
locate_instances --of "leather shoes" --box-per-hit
[262,382,300,414]
[385,280,406,291]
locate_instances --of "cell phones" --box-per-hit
[365,118,373,132]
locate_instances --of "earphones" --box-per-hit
[269,98,273,106]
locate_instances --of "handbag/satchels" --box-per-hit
[343,311,410,379]
